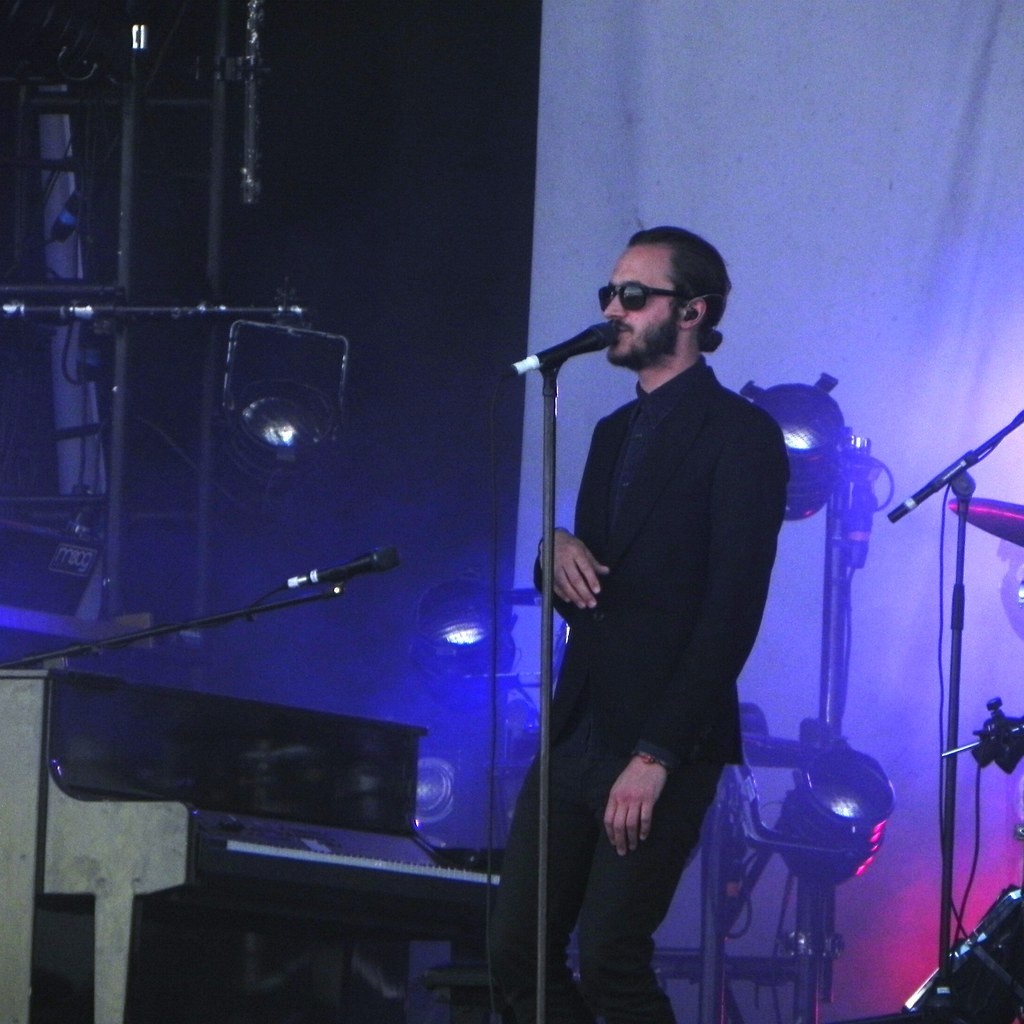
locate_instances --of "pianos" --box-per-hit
[1,667,512,1024]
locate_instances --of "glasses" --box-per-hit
[599,282,692,312]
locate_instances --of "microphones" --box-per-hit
[500,323,615,382]
[288,545,400,594]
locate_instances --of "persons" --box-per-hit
[479,226,789,1024]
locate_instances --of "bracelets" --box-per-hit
[634,750,671,773]
